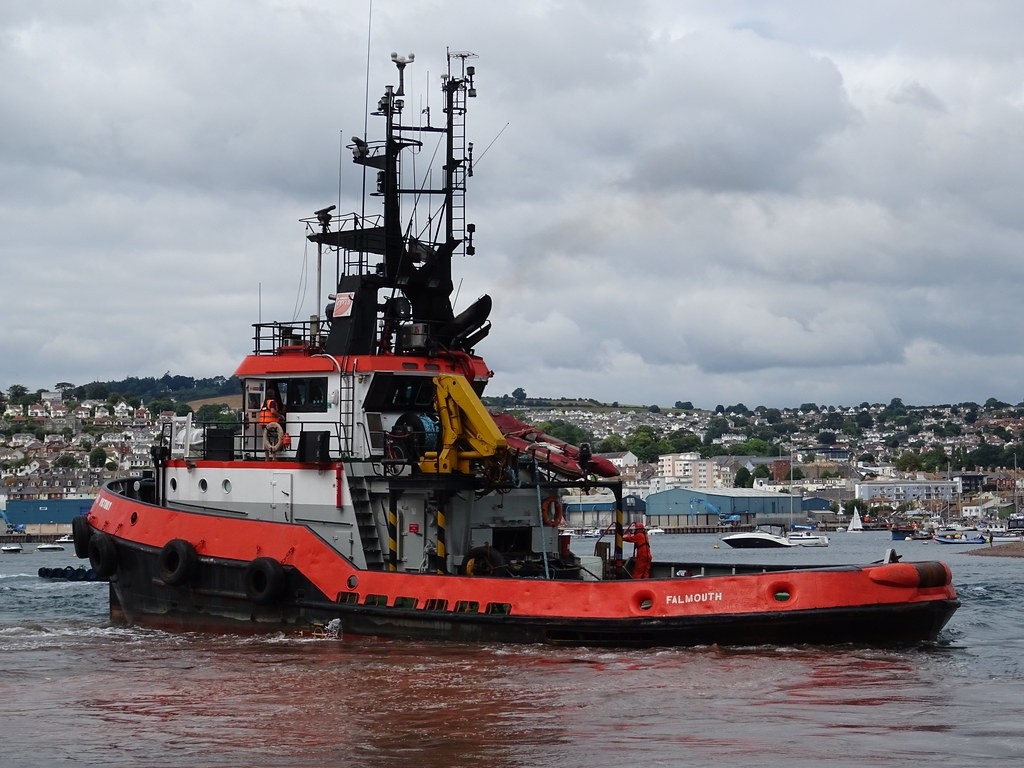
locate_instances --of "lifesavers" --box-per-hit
[264,422,283,451]
[542,497,562,526]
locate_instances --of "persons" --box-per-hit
[624,524,653,580]
[990,533,994,546]
[259,389,285,430]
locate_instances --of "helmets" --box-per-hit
[636,523,645,528]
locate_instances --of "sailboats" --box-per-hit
[847,507,864,534]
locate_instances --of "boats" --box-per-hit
[36,542,65,553]
[889,501,1024,545]
[718,532,829,549]
[646,526,667,536]
[584,529,601,538]
[71,2,960,653]
[2,543,23,553]
[56,535,74,542]
[556,529,579,538]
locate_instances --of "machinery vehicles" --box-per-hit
[0,509,25,534]
[688,497,741,527]
